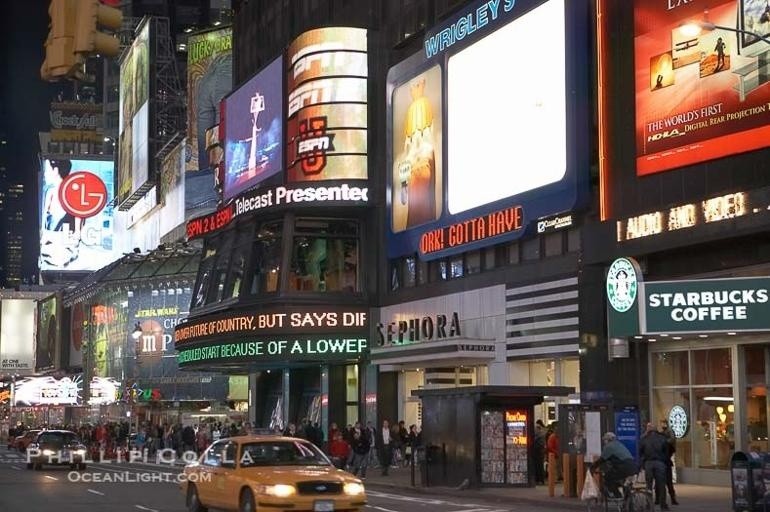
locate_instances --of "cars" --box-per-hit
[727,421,768,452]
[179,434,367,511]
[14,430,40,453]
[27,430,89,470]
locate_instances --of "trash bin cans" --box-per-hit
[416,445,446,487]
[729,449,770,512]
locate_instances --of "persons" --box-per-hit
[42,159,86,234]
[13,416,420,478]
[714,38,728,67]
[533,415,680,511]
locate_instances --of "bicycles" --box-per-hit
[585,469,653,512]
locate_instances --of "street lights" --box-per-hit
[10,374,17,433]
[132,320,143,420]
